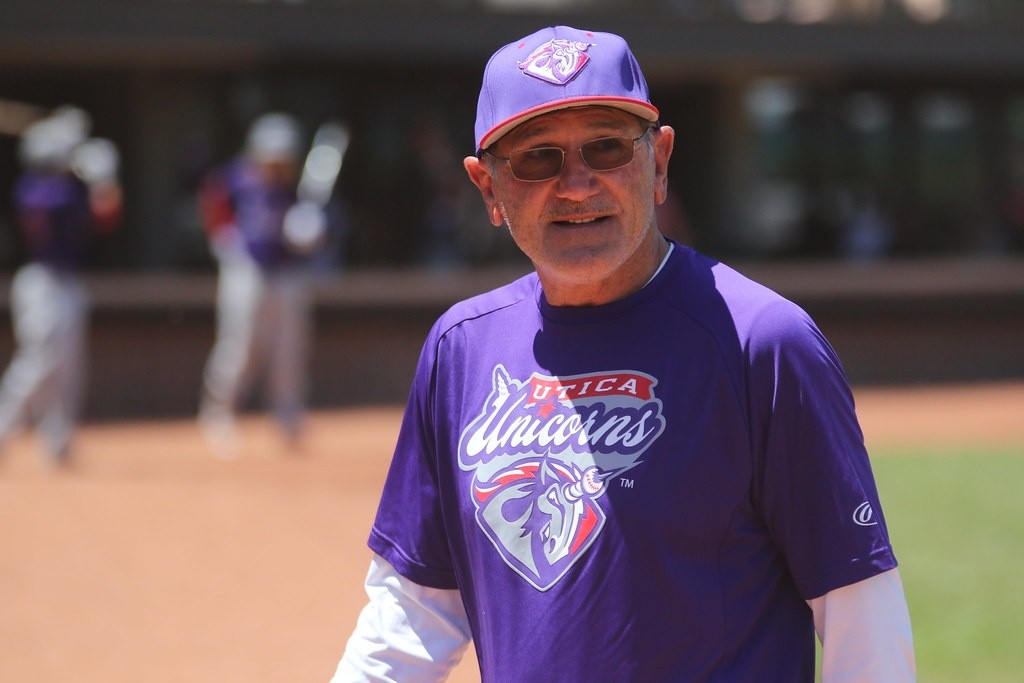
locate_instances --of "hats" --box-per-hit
[21,107,86,164]
[245,113,304,165]
[475,25,660,156]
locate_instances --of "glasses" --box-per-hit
[484,126,653,183]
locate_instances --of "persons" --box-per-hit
[0,109,118,476]
[196,116,317,461]
[327,26,915,683]
